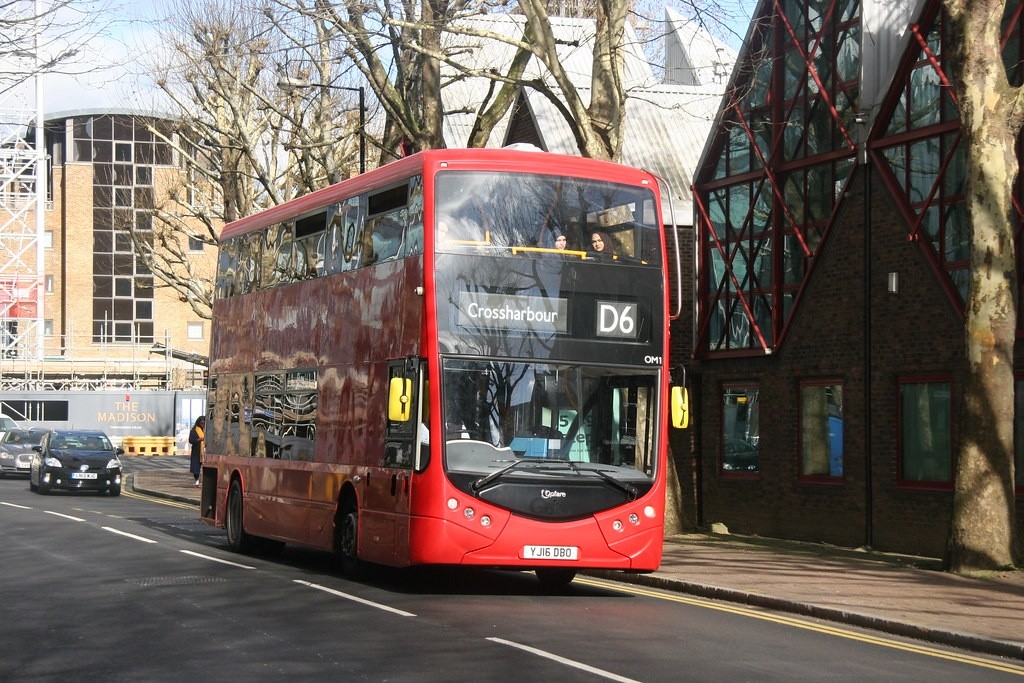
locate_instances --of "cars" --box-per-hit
[0,427,54,478]
[30,429,124,497]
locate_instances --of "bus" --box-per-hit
[200,143,688,590]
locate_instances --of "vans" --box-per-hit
[0,412,24,448]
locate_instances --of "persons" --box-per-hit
[14,435,21,442]
[589,228,617,253]
[53,435,68,448]
[547,227,569,250]
[188,416,206,486]
[85,436,97,448]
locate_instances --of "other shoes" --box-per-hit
[193,480,202,488]
